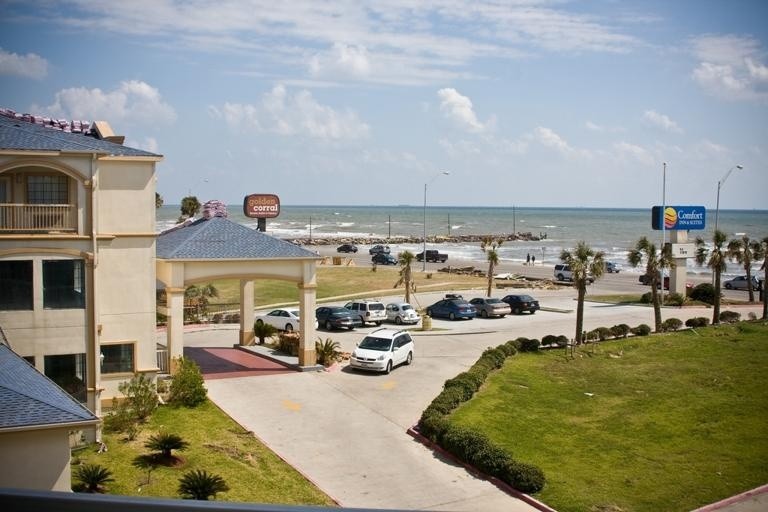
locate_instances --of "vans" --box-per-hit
[347,328,415,374]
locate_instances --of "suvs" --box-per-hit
[554,264,595,283]
[345,301,387,326]
[369,244,390,255]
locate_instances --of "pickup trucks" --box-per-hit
[601,260,622,273]
[415,249,448,263]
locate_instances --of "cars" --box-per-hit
[499,295,539,315]
[335,245,357,253]
[385,303,420,325]
[253,308,318,332]
[316,306,362,331]
[424,299,476,321]
[654,276,694,290]
[636,272,670,285]
[722,275,760,290]
[371,253,398,265]
[468,298,511,318]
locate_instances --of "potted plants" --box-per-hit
[313,336,341,367]
[253,322,277,345]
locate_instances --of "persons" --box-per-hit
[531,255,535,266]
[525,253,530,267]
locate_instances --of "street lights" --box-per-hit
[422,169,449,270]
[660,160,670,305]
[715,163,743,228]
